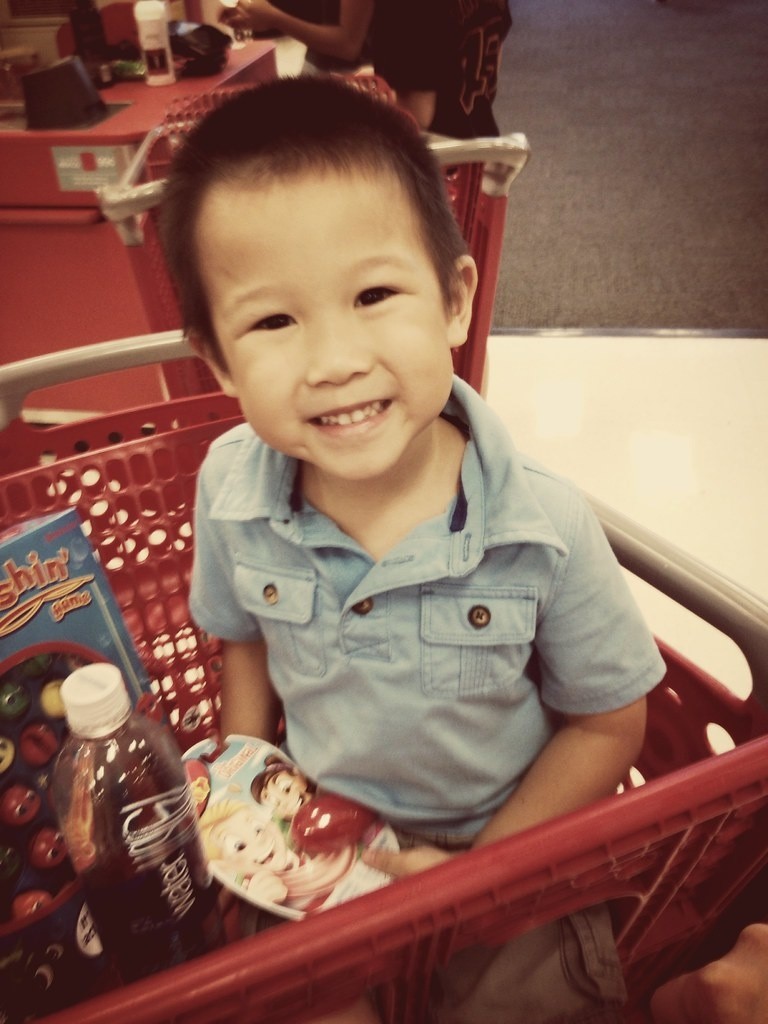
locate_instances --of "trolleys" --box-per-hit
[93,69,531,433]
[2,327,768,1024]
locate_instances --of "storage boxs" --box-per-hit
[0,506,164,723]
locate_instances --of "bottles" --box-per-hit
[53,661,227,982]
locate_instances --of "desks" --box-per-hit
[0,41,278,423]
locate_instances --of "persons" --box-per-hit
[220,0,512,138]
[159,78,670,1024]
